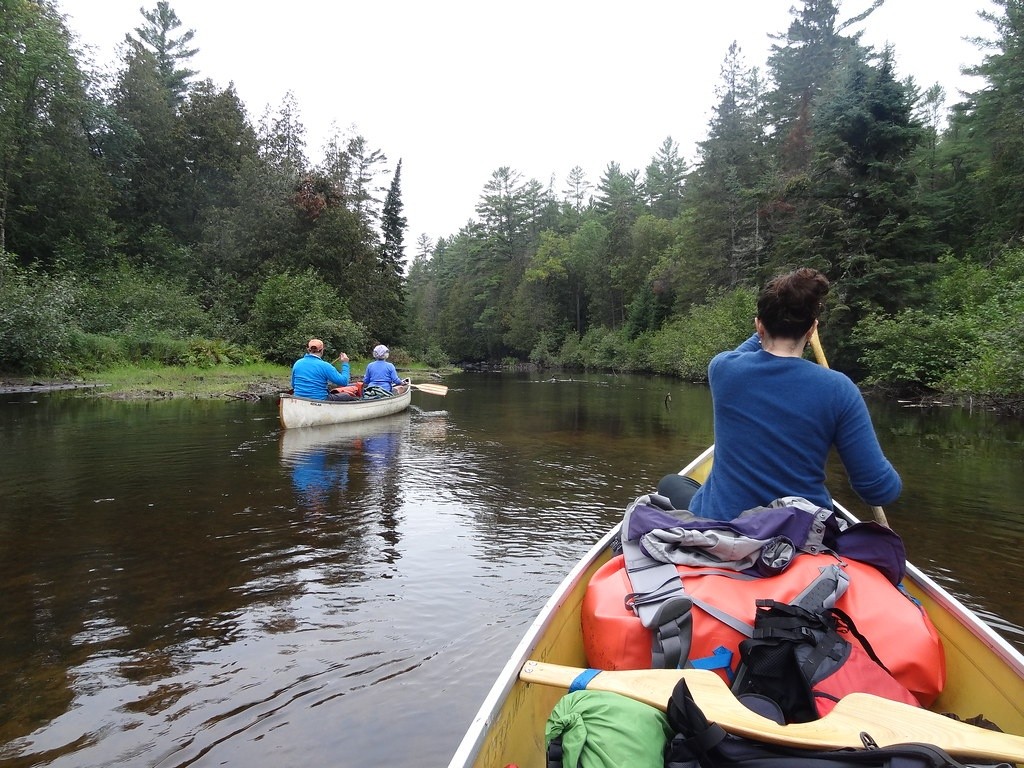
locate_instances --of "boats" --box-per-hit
[278,376,412,430]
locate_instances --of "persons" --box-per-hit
[292,339,351,401]
[657,269,903,522]
[364,345,404,392]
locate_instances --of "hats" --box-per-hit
[373,344,389,360]
[308,338,324,351]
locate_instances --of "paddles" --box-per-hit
[275,356,341,408]
[406,383,449,396]
[809,329,890,528]
